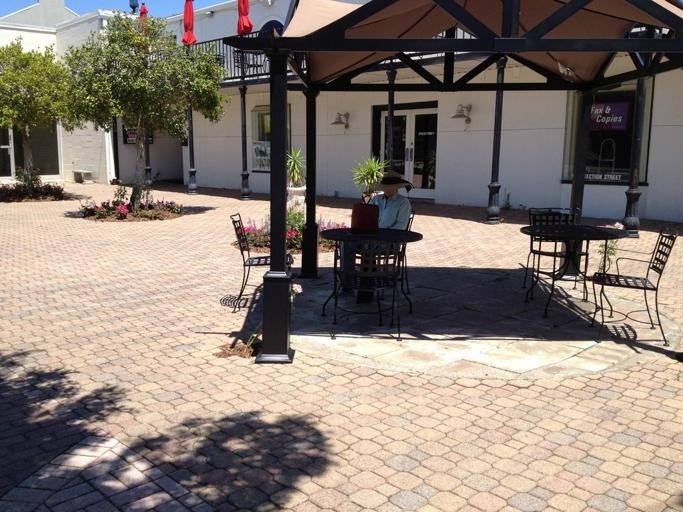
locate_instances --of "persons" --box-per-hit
[339,173,412,303]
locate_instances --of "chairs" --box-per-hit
[523,204,679,347]
[228,212,292,315]
[335,211,415,297]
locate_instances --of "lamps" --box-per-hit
[446,103,472,125]
[328,111,349,131]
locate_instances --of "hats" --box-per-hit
[375,172,412,189]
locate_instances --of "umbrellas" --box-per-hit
[181,0,197,54]
[236,0,253,34]
[138,3,149,24]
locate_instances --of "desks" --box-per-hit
[320,226,423,341]
[518,223,629,319]
